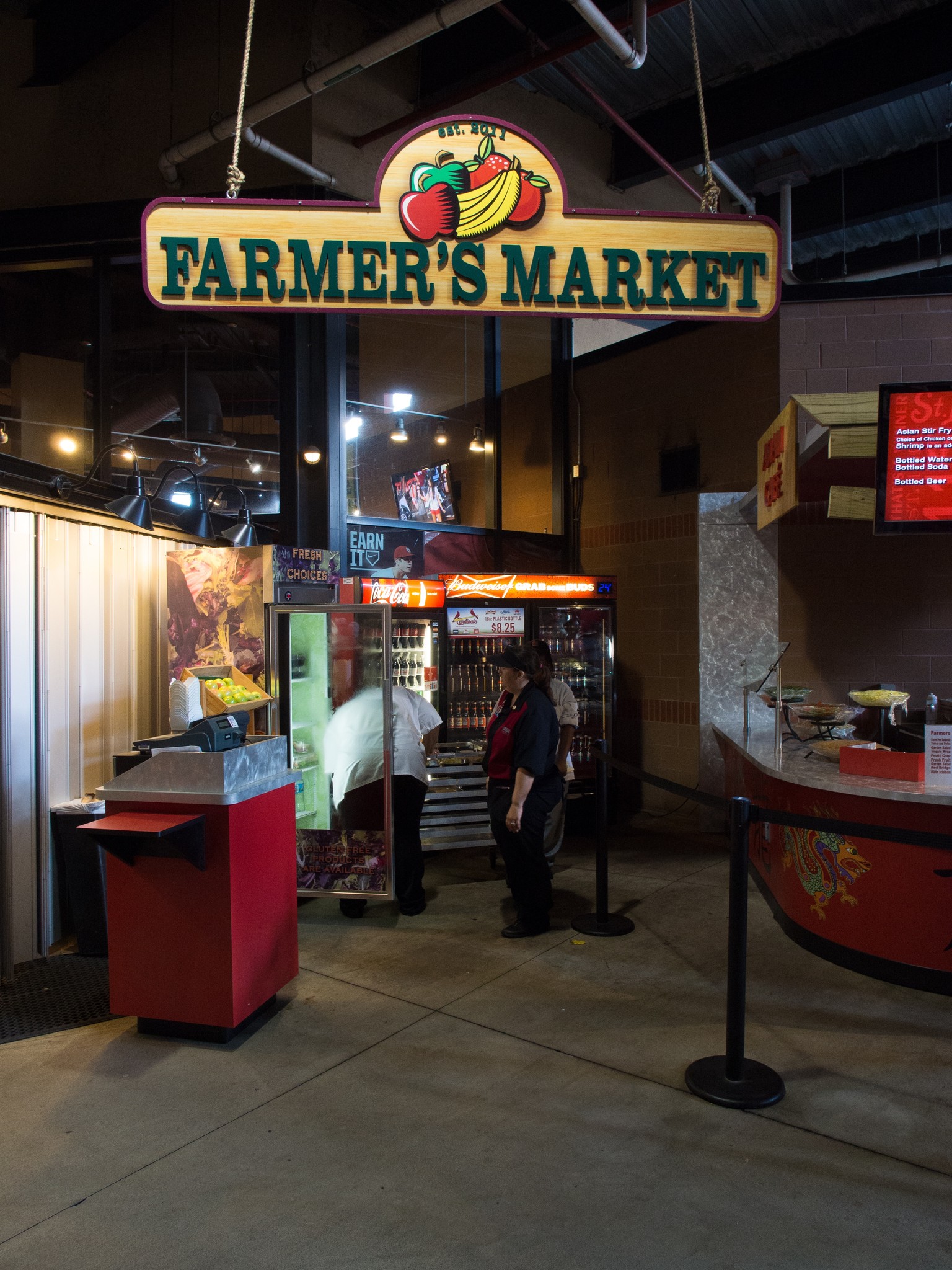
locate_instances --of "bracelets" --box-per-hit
[441,508,443,511]
[424,498,426,501]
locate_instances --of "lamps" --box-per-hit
[50,443,258,547]
[391,413,484,451]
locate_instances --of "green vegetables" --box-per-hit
[765,685,812,698]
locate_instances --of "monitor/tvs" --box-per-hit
[188,709,246,729]
[390,458,461,525]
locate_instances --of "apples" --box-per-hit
[205,678,261,705]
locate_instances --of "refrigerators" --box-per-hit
[417,572,617,853]
[167,545,394,900]
[330,576,445,747]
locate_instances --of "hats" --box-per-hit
[486,643,526,674]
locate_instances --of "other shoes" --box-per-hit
[340,899,367,918]
[395,888,426,916]
[513,902,549,914]
[501,919,551,938]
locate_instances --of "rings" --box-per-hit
[511,822,515,824]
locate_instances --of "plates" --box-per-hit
[757,694,776,705]
[786,702,848,718]
[809,745,891,762]
[828,727,856,736]
[835,708,867,722]
[848,692,911,706]
[763,689,816,699]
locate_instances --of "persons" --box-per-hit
[481,644,563,939]
[323,687,443,918]
[527,639,579,878]
[411,477,446,523]
[370,545,415,579]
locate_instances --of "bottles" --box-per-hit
[363,652,424,688]
[577,702,592,727]
[295,779,306,813]
[539,625,583,653]
[447,657,502,694]
[553,662,589,689]
[449,700,497,732]
[925,704,937,724]
[926,693,937,704]
[292,652,306,679]
[570,735,593,763]
[362,621,426,650]
[449,638,513,656]
